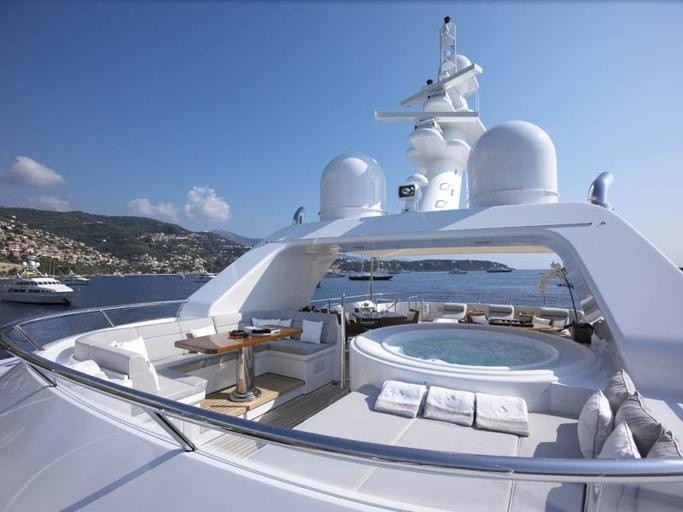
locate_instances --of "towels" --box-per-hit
[374,378,530,438]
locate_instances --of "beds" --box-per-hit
[260,384,682,511]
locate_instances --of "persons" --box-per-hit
[441,16,452,33]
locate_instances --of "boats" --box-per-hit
[194,269,219,283]
[449,269,468,274]
[0,258,90,304]
[486,266,512,273]
[349,271,400,281]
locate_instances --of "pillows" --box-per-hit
[553,320,566,328]
[114,336,150,362]
[281,318,292,341]
[577,390,614,458]
[191,326,217,354]
[303,319,324,344]
[606,369,634,399]
[252,317,280,326]
[596,422,641,511]
[617,393,661,446]
[643,430,682,510]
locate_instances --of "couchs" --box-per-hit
[76,312,339,406]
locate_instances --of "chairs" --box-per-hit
[341,303,420,331]
[539,307,569,331]
[436,302,468,323]
[488,305,514,323]
